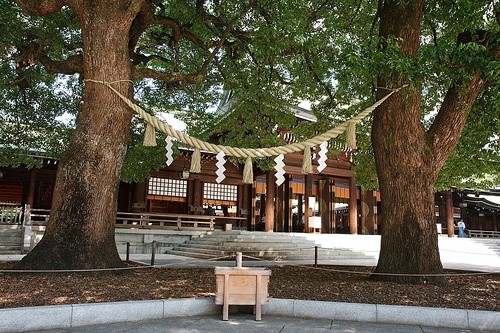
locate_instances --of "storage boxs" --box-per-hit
[214,265,272,321]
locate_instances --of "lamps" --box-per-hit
[178,157,190,180]
[458,193,467,209]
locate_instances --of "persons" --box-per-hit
[458,218,467,238]
[453,218,458,235]
[262,213,266,231]
[203,202,216,222]
[292,213,298,232]
[301,213,305,232]
